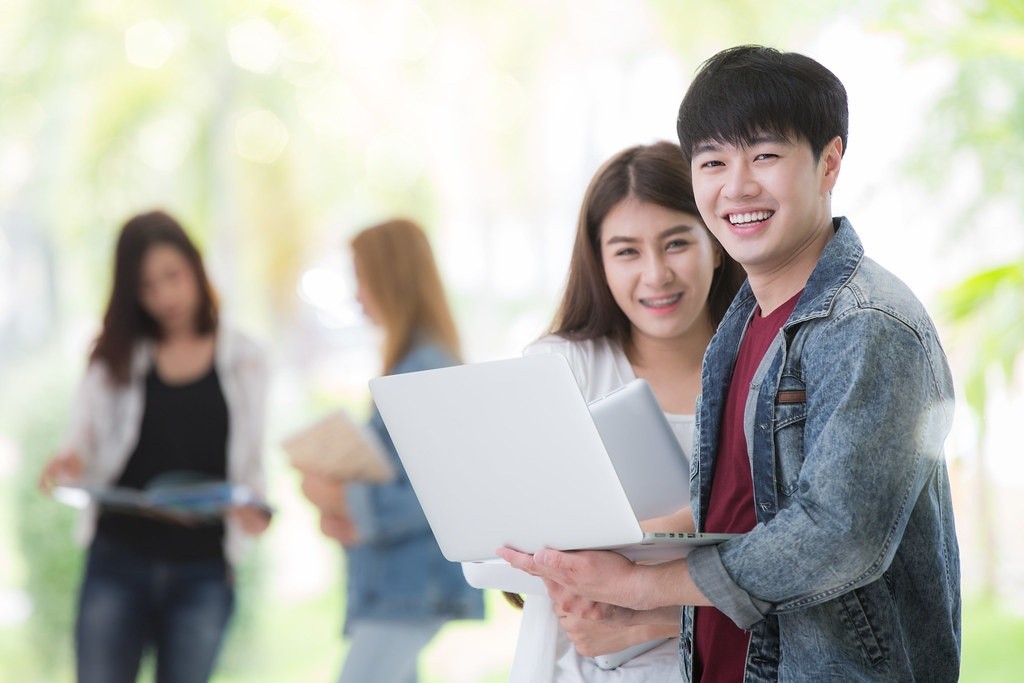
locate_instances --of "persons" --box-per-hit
[461,142,747,683]
[285,221,485,683]
[40,213,273,683]
[496,48,961,683]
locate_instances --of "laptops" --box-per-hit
[368,352,743,562]
[587,379,693,672]
